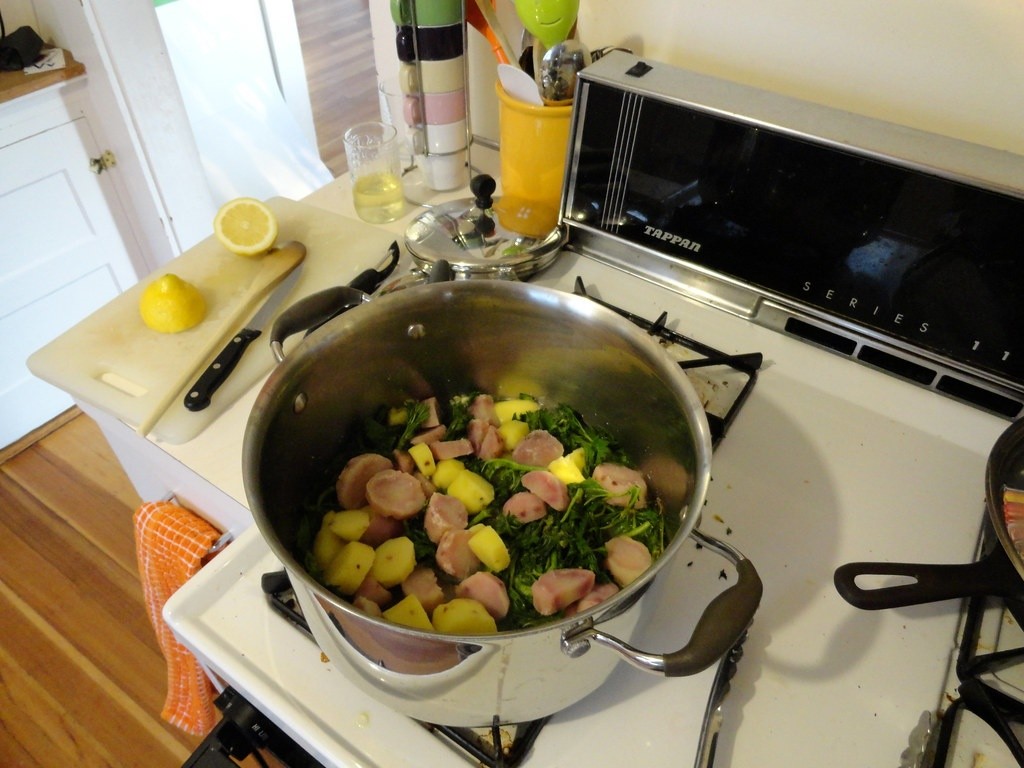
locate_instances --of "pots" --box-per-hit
[400,173,572,288]
[833,415,1022,632]
[242,285,765,730]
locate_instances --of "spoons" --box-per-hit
[464,2,604,108]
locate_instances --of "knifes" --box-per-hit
[182,261,309,413]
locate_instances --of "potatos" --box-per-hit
[295,389,670,634]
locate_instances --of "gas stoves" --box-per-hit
[159,47,1023,767]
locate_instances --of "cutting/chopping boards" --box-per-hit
[25,195,417,508]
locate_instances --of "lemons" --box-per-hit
[213,197,279,257]
[139,274,209,332]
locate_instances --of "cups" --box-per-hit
[388,0,474,192]
[378,76,424,155]
[496,78,576,240]
[342,121,408,224]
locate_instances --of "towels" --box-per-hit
[130,500,233,739]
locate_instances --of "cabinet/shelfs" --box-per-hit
[0,76,148,465]
